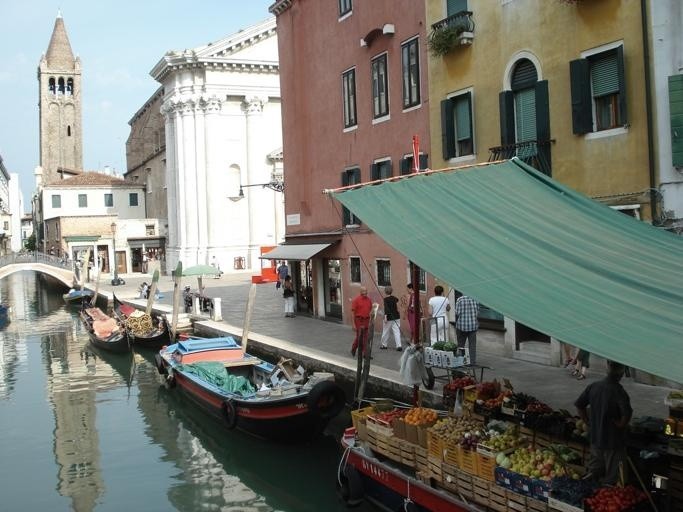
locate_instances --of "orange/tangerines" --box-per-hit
[399,406,437,427]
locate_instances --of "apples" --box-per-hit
[483,432,580,482]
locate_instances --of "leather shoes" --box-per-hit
[380,346,387,349]
[397,347,402,351]
[572,370,579,375]
[577,374,585,380]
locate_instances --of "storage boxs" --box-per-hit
[392,417,427,447]
[425,347,470,368]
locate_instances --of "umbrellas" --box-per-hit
[183,265,223,276]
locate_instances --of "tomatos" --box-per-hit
[584,484,647,511]
[444,377,474,391]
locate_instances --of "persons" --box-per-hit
[427,286,450,347]
[378,286,402,351]
[183,285,213,312]
[574,359,633,488]
[137,282,159,299]
[570,349,590,380]
[406,283,415,344]
[282,275,296,318]
[276,261,287,289]
[349,287,373,360]
[453,293,479,366]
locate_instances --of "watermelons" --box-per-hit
[432,340,457,353]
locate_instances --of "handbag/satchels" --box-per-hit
[284,289,293,298]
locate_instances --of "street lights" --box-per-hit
[110,222,118,278]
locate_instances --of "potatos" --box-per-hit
[430,415,485,446]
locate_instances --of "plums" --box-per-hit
[370,409,409,425]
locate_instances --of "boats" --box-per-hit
[110,291,168,342]
[62,290,95,306]
[155,332,346,441]
[156,385,342,512]
[84,341,136,407]
[323,133,683,512]
[78,308,129,352]
